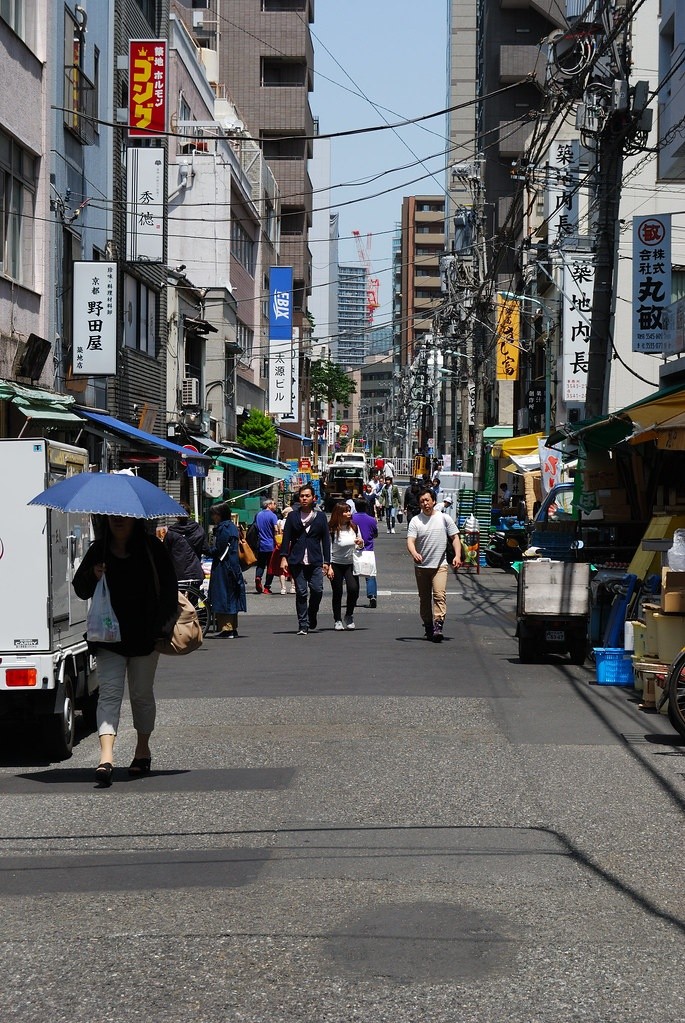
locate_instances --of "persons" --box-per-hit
[407,488,462,642]
[163,501,209,606]
[255,498,301,594]
[363,455,402,533]
[498,483,511,505]
[404,458,453,525]
[72,516,178,786]
[280,485,330,635]
[327,502,364,629]
[208,502,247,639]
[352,497,378,607]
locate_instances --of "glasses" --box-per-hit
[270,503,277,505]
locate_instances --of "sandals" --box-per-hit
[95,763,112,787]
[128,758,151,774]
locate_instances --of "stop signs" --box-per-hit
[339,424,349,434]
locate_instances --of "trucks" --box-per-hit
[322,451,372,513]
[0,436,97,765]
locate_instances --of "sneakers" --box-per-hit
[343,616,355,629]
[334,621,345,630]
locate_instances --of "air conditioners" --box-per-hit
[183,377,199,406]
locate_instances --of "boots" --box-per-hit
[422,621,435,641]
[434,620,444,642]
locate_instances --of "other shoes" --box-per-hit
[370,598,377,608]
[387,529,390,533]
[215,630,238,638]
[308,628,319,632]
[289,589,296,593]
[391,528,395,533]
[281,588,286,595]
[264,589,274,594]
[255,578,261,592]
[297,631,307,635]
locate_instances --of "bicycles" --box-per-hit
[174,578,213,639]
[658,645,685,728]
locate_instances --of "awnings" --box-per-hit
[277,428,313,446]
[212,455,296,503]
[13,403,87,444]
[545,385,685,468]
[233,447,291,469]
[491,431,544,475]
[76,408,213,459]
[184,436,227,472]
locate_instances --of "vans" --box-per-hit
[432,470,473,522]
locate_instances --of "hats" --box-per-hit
[377,455,381,457]
[444,497,453,503]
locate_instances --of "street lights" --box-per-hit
[438,368,459,472]
[419,401,438,457]
[498,290,551,439]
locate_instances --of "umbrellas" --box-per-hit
[27,473,190,568]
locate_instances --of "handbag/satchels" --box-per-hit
[397,510,403,523]
[353,547,377,577]
[171,591,204,655]
[237,531,257,572]
[86,575,121,642]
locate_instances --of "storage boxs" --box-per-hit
[522,452,635,686]
[225,489,262,524]
[660,564,685,612]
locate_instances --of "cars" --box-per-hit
[517,482,646,563]
